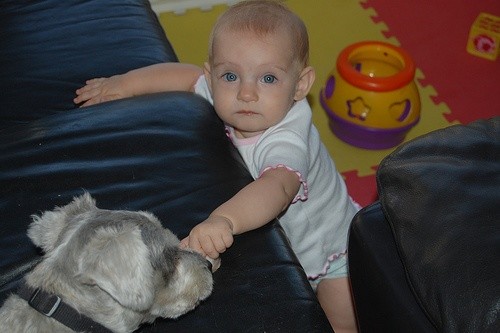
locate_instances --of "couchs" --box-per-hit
[0,0,335,333]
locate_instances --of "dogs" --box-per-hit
[0,187,216,333]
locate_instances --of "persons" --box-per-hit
[73,0,363,333]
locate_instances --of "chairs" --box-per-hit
[345,113,500,333]
